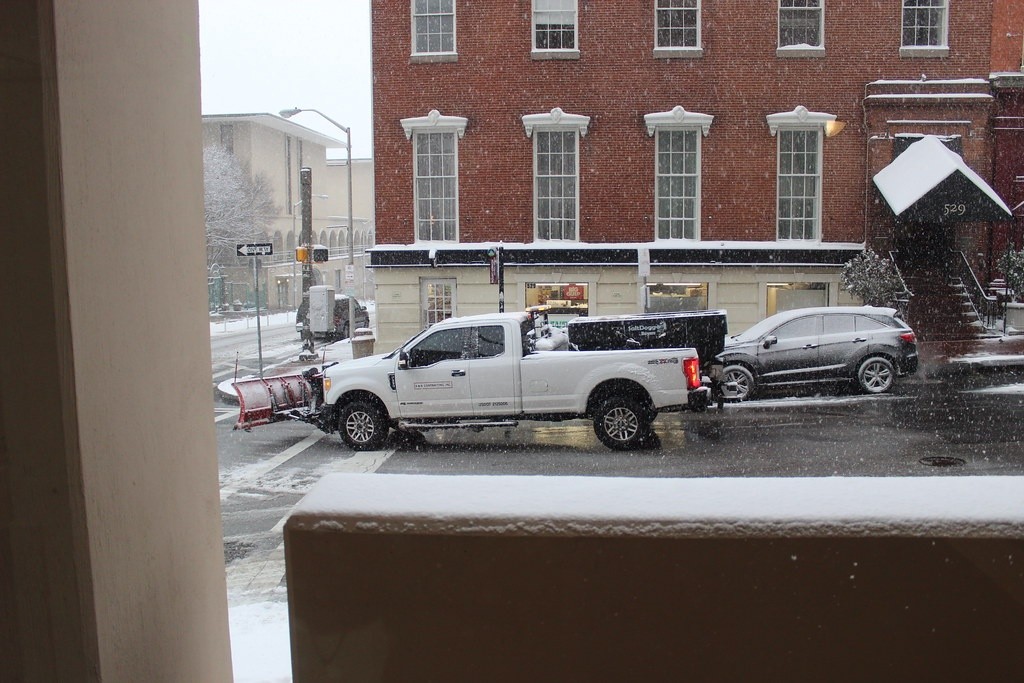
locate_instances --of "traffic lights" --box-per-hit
[295,247,307,262]
[313,246,328,262]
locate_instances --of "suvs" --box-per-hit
[296,294,369,341]
[714,303,918,403]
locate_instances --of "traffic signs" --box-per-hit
[236,244,273,256]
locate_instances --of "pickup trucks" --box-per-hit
[229,312,719,453]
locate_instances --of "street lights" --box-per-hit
[293,194,329,312]
[280,108,355,343]
